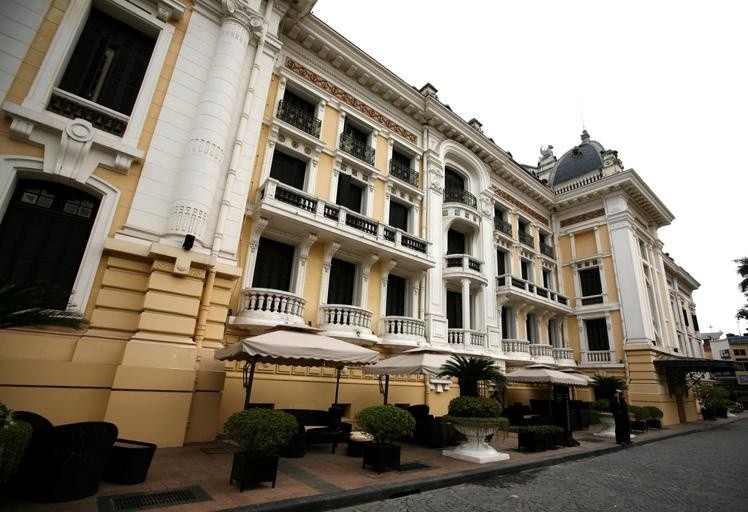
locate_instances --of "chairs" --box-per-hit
[249,401,342,454]
[407,405,445,449]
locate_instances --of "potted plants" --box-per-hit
[442,396,566,464]
[225,405,298,492]
[695,385,729,420]
[353,405,417,473]
[591,399,663,438]
